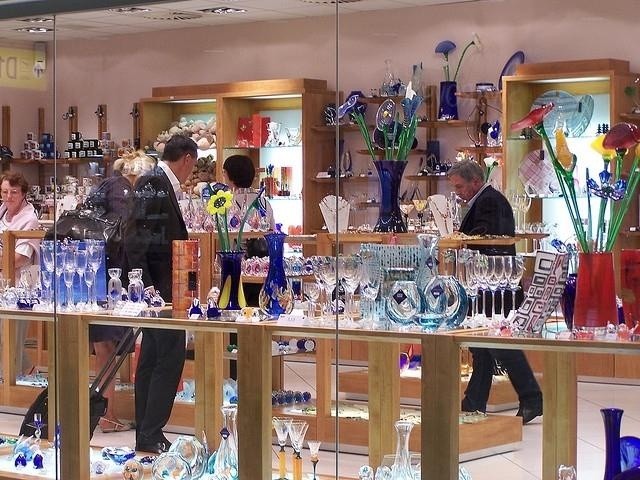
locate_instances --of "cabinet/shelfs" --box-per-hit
[312,84,503,368]
[218,78,343,365]
[501,58,640,383]
[131,84,219,199]
[0,311,639,480]
[0,102,137,232]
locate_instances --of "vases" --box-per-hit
[216,250,248,322]
[259,235,295,318]
[620,248,640,341]
[436,81,458,120]
[373,160,410,234]
[573,252,619,339]
[600,408,624,480]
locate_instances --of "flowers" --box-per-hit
[338,81,425,161]
[200,181,267,252]
[510,102,640,254]
[434,32,483,82]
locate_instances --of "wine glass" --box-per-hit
[453,246,524,328]
[508,191,532,234]
[300,253,382,329]
[0,236,103,315]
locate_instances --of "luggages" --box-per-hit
[18,326,144,442]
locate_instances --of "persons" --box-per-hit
[0,171,39,376]
[122,136,198,452]
[447,159,541,423]
[217,154,275,229]
[85,146,155,434]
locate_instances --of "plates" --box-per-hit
[520,87,596,196]
[497,51,525,92]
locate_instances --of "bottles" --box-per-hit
[213,404,239,480]
[562,273,577,329]
[391,421,415,479]
[176,185,271,233]
[600,407,625,480]
[416,231,445,316]
[382,59,394,94]
[106,265,165,313]
[259,233,295,318]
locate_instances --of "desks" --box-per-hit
[0,311,639,480]
[0,300,639,479]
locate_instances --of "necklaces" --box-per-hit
[58,201,74,215]
[432,200,451,233]
[322,201,350,211]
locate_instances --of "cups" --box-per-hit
[20,130,134,159]
[24,164,105,211]
[389,279,419,320]
[382,450,422,479]
[150,435,207,480]
[179,374,239,405]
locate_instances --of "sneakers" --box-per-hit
[135,433,172,454]
[516,401,546,424]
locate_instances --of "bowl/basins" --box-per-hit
[107,451,135,464]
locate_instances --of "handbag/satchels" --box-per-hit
[44,177,124,260]
